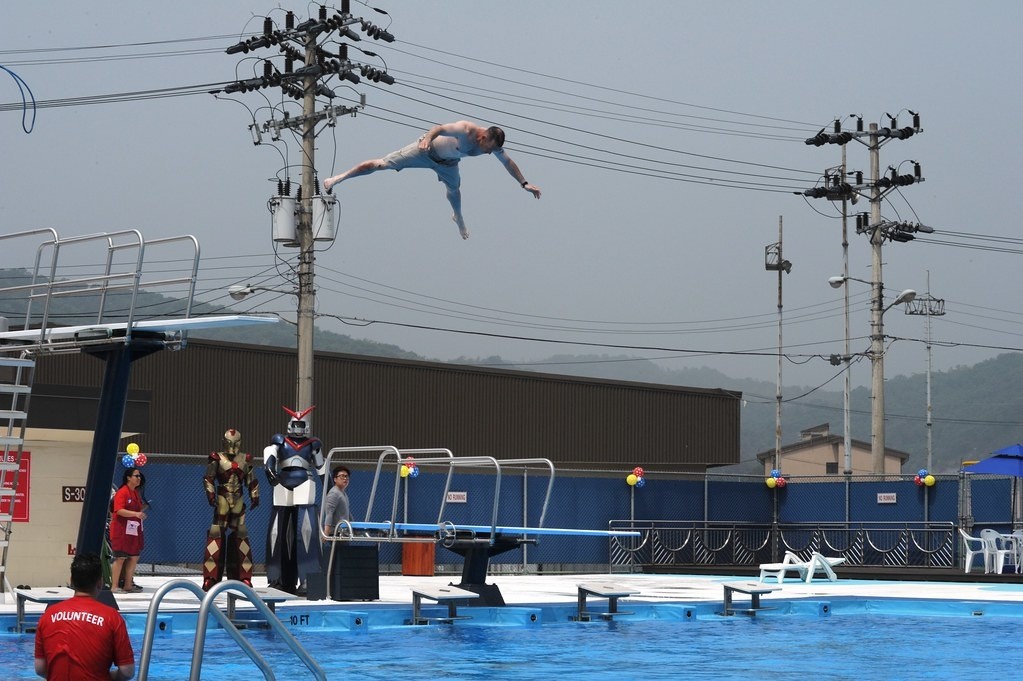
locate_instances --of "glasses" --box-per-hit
[335,474,350,480]
[130,475,141,478]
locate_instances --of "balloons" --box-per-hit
[626,474,637,485]
[126,443,139,455]
[914,469,935,487]
[135,453,147,467]
[636,477,646,488]
[122,455,134,468]
[766,478,776,488]
[770,470,781,478]
[633,467,643,477]
[406,457,416,468]
[409,466,419,478]
[777,478,786,487]
[401,466,409,478]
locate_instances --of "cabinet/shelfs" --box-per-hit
[401,534,435,577]
[306,544,380,602]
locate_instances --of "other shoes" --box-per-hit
[123,586,142,593]
[111,587,127,594]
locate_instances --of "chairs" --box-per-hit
[759,550,847,583]
[958,528,1023,575]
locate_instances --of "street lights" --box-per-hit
[828,276,916,481]
[227,285,314,435]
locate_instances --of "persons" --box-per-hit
[321,466,358,555]
[324,121,541,239]
[262,404,330,597]
[34,554,135,681]
[109,468,149,593]
[203,428,259,591]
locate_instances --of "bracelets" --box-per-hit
[521,181,528,188]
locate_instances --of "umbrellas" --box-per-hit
[961,443,1023,542]
